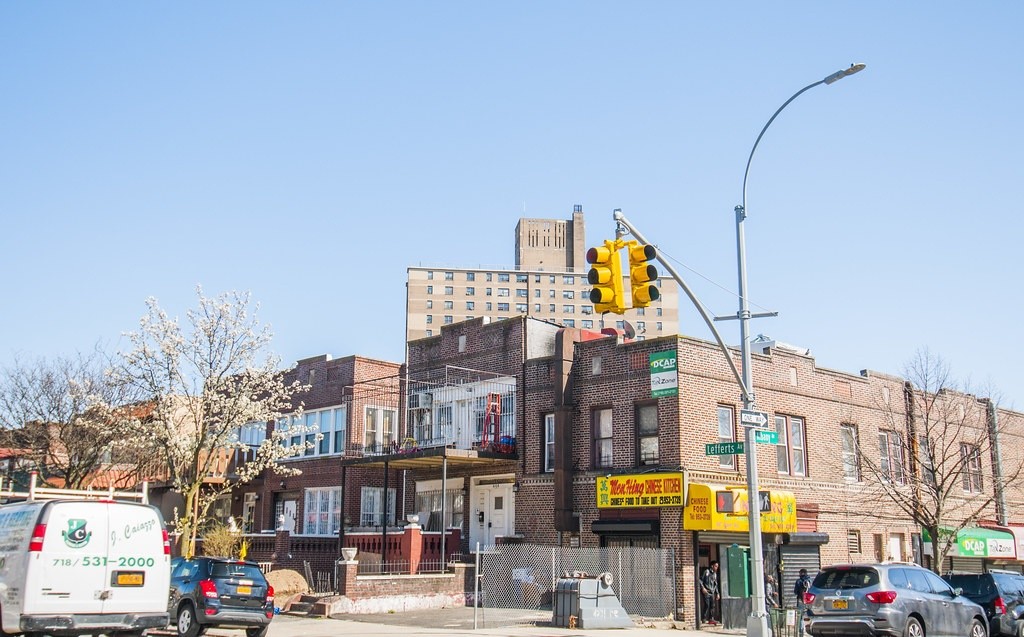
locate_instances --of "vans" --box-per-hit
[0,494,175,637]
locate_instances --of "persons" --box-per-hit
[793,568,812,637]
[697,560,721,624]
[765,574,779,628]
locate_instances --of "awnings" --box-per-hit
[922,525,1016,559]
[979,523,1024,564]
[683,483,798,533]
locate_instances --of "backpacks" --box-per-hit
[801,579,811,592]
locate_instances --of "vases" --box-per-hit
[407,514,419,527]
[341,546,358,561]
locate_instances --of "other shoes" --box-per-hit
[709,619,720,624]
[703,619,709,623]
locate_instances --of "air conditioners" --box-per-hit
[408,393,432,410]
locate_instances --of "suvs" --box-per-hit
[798,560,990,637]
[940,568,1024,637]
[161,554,276,637]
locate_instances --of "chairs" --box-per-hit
[842,576,859,589]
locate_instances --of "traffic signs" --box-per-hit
[705,441,744,456]
[740,409,769,430]
[754,430,779,445]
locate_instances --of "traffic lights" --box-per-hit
[627,242,660,308]
[585,242,626,315]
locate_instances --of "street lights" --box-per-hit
[735,62,870,637]
